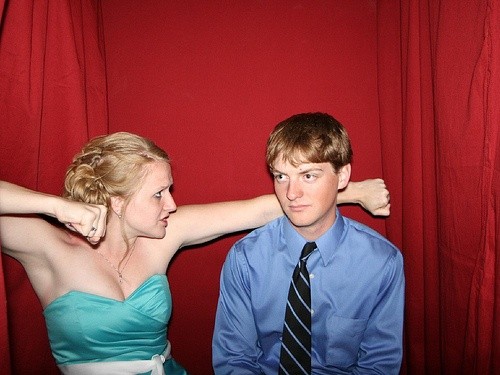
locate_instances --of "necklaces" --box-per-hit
[94,239,136,284]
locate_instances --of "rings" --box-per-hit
[91,227,97,232]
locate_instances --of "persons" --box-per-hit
[1,130,392,375]
[211,113,408,375]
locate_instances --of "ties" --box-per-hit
[278,242,318,375]
